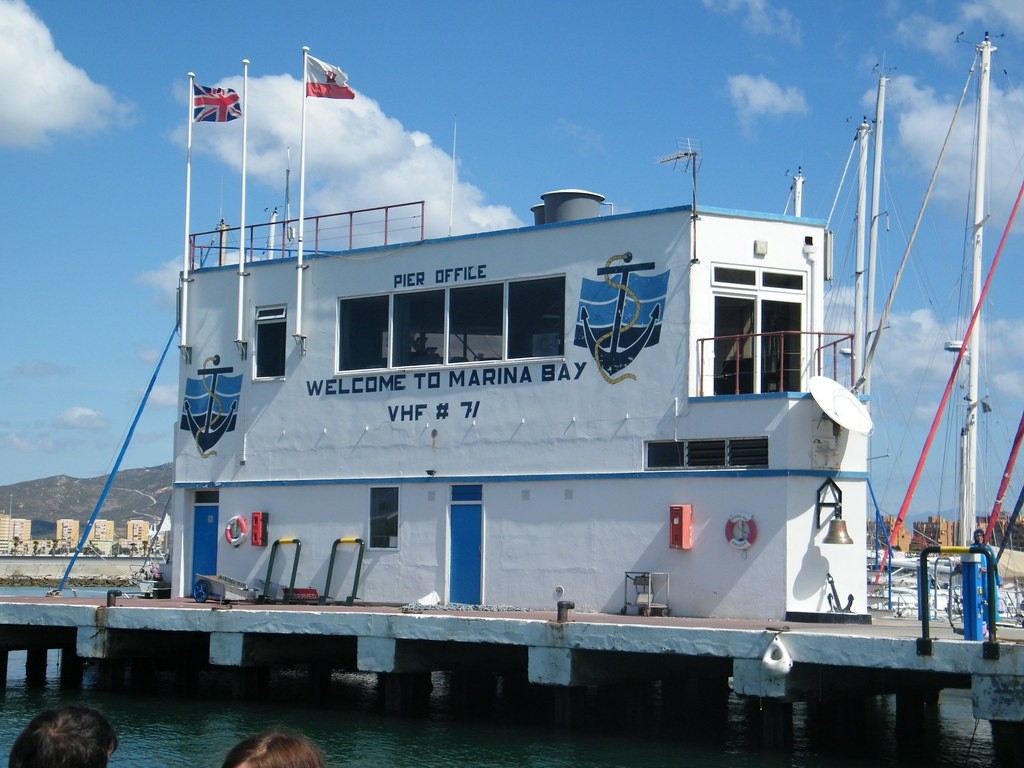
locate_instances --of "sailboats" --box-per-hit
[775,28,1023,628]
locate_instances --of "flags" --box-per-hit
[192,80,243,123]
[304,53,355,100]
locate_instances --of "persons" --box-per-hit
[7,705,119,768]
[219,733,326,768]
[954,530,1001,639]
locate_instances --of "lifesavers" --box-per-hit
[223,516,248,546]
[723,514,758,550]
